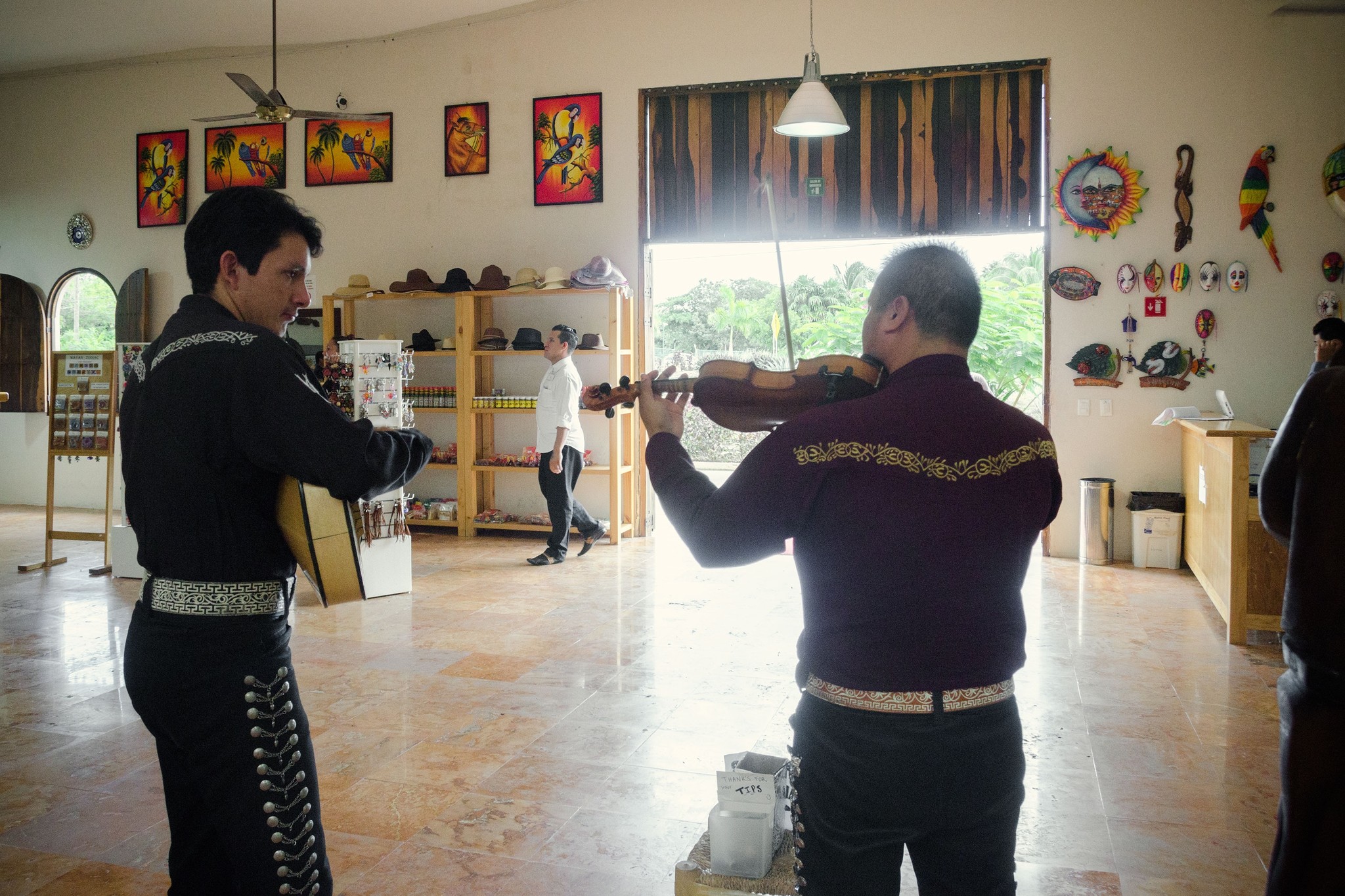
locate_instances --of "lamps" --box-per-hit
[773,0,851,137]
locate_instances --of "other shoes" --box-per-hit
[578,528,607,557]
[527,551,562,565]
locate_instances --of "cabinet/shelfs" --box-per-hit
[1176,408,1286,645]
[330,338,414,598]
[319,290,466,539]
[464,278,636,545]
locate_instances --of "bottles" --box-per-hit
[402,386,456,408]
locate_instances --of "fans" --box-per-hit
[190,0,391,122]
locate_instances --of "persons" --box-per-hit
[638,244,1063,896]
[527,324,607,566]
[313,334,364,380]
[116,186,434,896]
[1257,311,1345,896]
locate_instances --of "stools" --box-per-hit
[674,829,798,896]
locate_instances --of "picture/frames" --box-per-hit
[533,92,603,206]
[205,123,287,193]
[135,130,188,227]
[303,112,393,186]
[444,102,490,177]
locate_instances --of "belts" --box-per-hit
[138,575,297,617]
[806,670,1015,714]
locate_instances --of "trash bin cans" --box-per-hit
[1078,477,1114,565]
[1130,489,1185,570]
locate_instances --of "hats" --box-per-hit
[389,268,441,293]
[477,327,509,345]
[405,329,442,351]
[575,333,609,350]
[435,337,456,351]
[506,268,542,289]
[538,281,569,290]
[332,274,385,298]
[473,265,511,291]
[474,341,507,351]
[537,266,570,289]
[570,255,628,291]
[505,280,541,293]
[511,328,545,351]
[437,268,476,293]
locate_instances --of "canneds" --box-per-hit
[473,396,538,409]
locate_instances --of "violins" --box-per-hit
[582,354,884,419]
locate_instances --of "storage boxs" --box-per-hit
[703,749,793,877]
